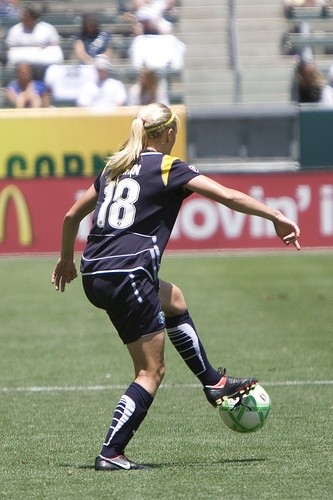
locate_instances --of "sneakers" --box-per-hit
[93,455,154,472]
[203,365,260,405]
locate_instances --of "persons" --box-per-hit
[5,1,63,64]
[126,68,169,106]
[280,0,333,55]
[290,46,326,102]
[128,8,185,71]
[72,9,111,82]
[52,103,300,471]
[133,0,172,11]
[7,61,51,108]
[75,55,126,107]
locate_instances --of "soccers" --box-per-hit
[219,383,271,434]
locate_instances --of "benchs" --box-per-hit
[283,5,333,101]
[0,9,184,109]
